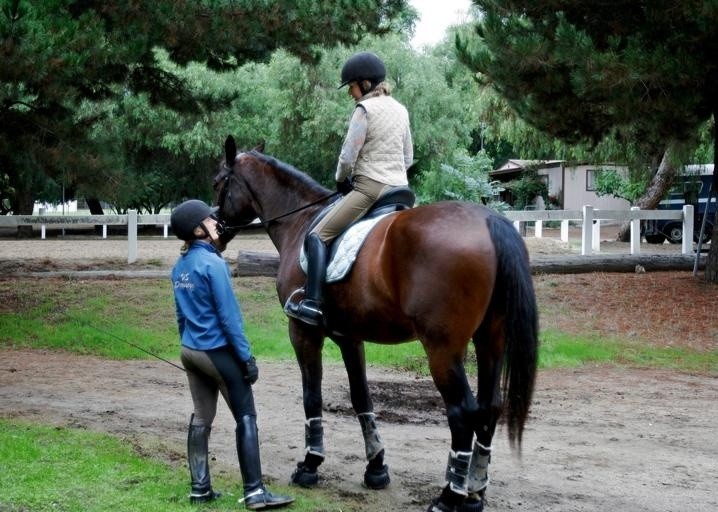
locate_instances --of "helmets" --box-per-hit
[337,52,386,90]
[170,200,220,240]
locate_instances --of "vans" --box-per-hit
[638,164,715,243]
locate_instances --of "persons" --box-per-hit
[284,52,416,325]
[168,198,297,510]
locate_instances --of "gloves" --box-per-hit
[239,354,260,385]
[335,177,352,195]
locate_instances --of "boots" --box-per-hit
[187,415,223,505]
[236,413,295,510]
[284,230,329,325]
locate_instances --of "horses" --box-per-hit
[209,134,539,512]
